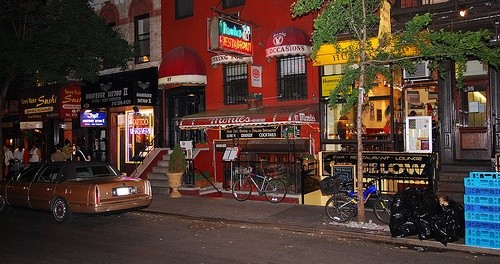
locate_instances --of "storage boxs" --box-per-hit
[463,171,500,249]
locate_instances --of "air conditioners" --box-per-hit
[402,59,432,77]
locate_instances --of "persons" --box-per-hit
[136,134,141,143]
[51,139,72,162]
[3,144,41,179]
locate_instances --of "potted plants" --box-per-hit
[166,144,186,198]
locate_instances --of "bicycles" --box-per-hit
[325,176,393,226]
[232,165,288,204]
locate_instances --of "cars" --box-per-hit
[0,161,153,225]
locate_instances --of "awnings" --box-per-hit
[265,26,312,63]
[158,46,208,85]
[313,31,436,67]
[81,66,158,110]
[179,103,320,130]
[210,52,254,68]
[17,84,82,119]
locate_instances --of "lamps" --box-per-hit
[460,2,495,18]
